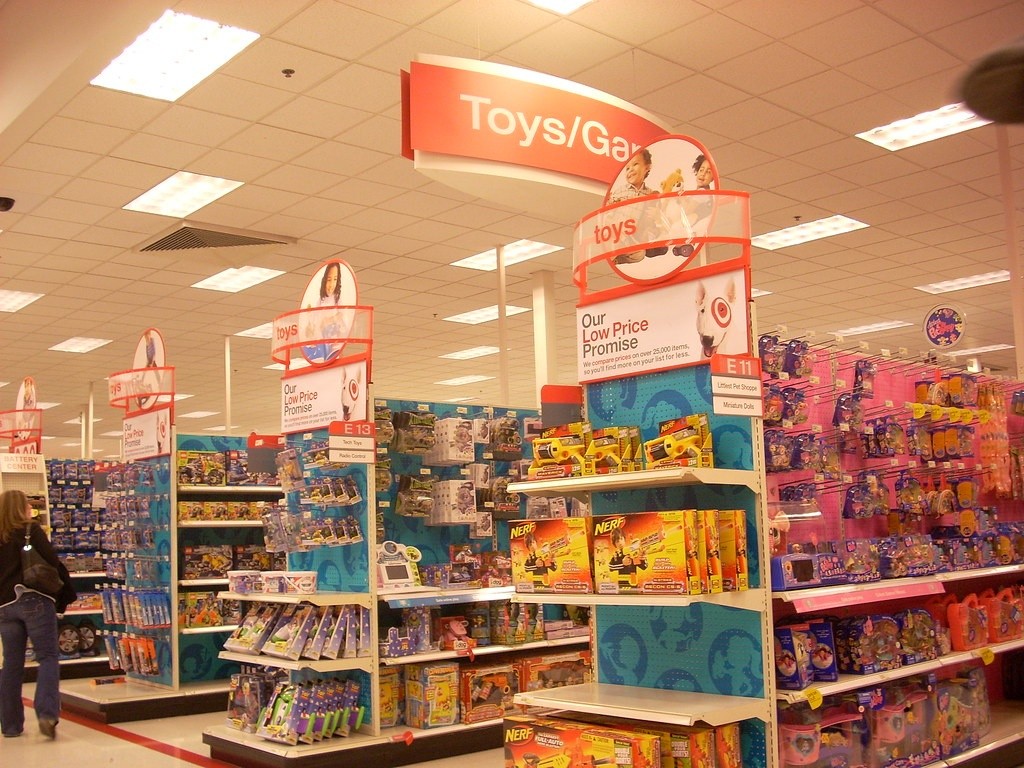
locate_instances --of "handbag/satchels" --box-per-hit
[20,522,65,594]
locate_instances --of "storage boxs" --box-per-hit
[175,406,1024,768]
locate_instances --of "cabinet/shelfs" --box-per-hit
[0,453,119,683]
[199,395,746,768]
[62,426,286,724]
[506,295,1024,768]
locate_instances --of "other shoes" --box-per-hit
[4,727,24,737]
[39,719,60,738]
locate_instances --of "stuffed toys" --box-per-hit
[659,168,698,232]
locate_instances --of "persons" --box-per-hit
[608,527,649,591]
[0,489,63,741]
[140,330,162,386]
[608,148,713,265]
[305,262,346,349]
[22,377,36,422]
[524,531,557,589]
